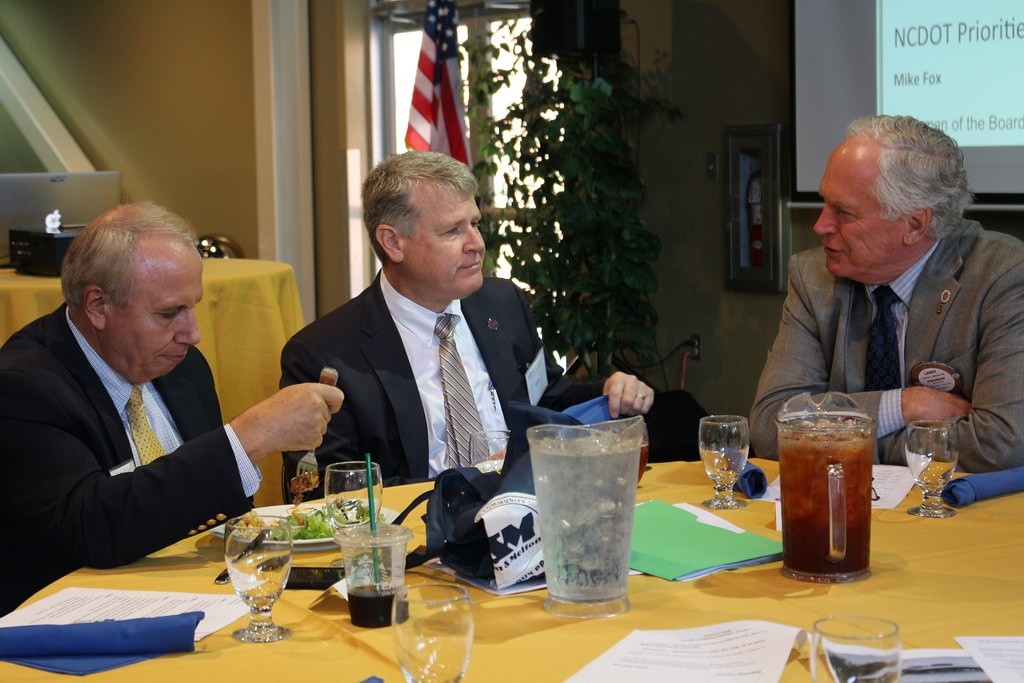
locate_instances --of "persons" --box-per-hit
[279,149,655,507]
[0,200,345,619]
[746,115,1024,475]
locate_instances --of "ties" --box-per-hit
[434,314,490,468]
[124,385,167,465]
[863,284,902,392]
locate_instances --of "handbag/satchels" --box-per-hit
[380,400,593,589]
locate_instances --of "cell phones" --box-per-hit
[285,567,346,590]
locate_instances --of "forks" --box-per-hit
[297,367,338,482]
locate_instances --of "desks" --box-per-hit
[0,256,306,508]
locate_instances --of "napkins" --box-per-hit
[0,611,205,676]
[722,451,769,499]
[940,466,1024,509]
[560,395,623,425]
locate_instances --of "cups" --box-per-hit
[334,524,412,628]
[391,584,473,683]
[527,415,644,618]
[809,617,901,682]
[638,423,648,485]
[469,429,510,474]
[777,391,876,584]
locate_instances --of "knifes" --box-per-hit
[215,529,268,585]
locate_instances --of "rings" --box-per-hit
[635,393,645,400]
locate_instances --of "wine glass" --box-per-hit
[225,514,293,643]
[698,415,750,510]
[905,420,959,519]
[324,460,383,566]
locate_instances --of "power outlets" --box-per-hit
[690,334,701,361]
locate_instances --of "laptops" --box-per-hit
[0,172,122,265]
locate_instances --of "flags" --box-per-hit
[406,0,474,172]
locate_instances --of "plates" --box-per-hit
[209,502,401,551]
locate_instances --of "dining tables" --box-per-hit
[0,457,1024,683]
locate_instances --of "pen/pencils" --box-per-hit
[213,530,268,586]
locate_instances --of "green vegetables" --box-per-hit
[261,500,387,540]
